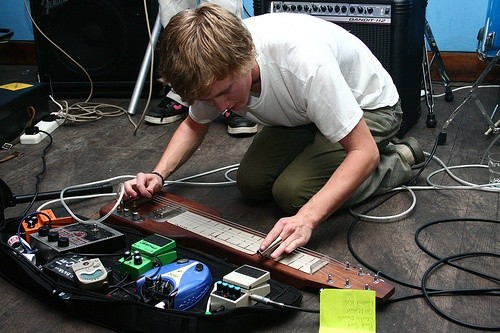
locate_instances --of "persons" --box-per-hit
[122,3,426,259]
[143,0,258,134]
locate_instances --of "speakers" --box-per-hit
[28,0,163,98]
[252,0,428,133]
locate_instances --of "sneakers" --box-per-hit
[227,112,258,134]
[144,96,188,124]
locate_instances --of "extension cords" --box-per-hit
[20,111,67,145]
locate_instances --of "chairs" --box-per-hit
[438,0,500,145]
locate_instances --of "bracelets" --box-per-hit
[151,172,164,185]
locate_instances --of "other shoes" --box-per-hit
[387,136,426,166]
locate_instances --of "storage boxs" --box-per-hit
[0,83,51,150]
